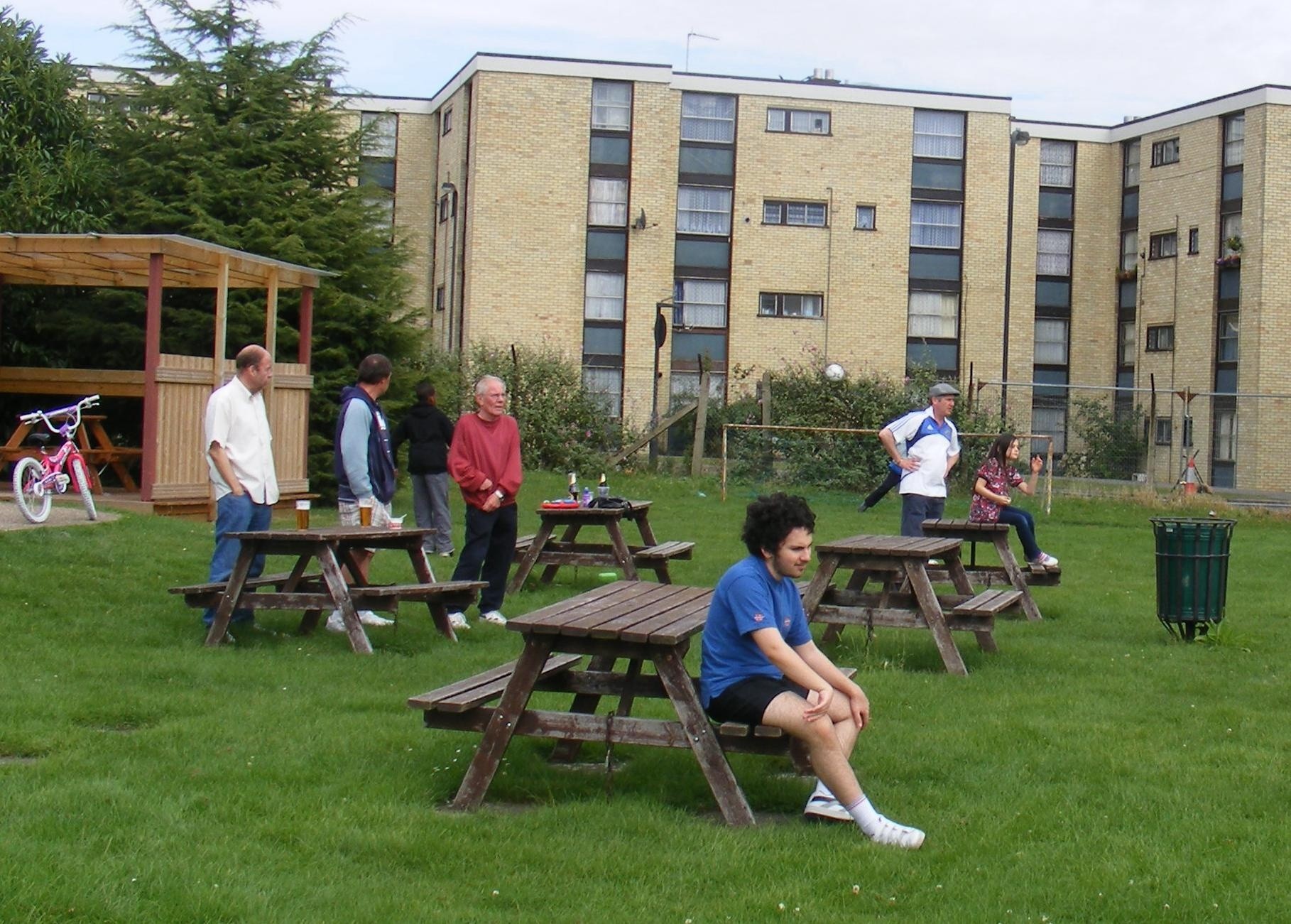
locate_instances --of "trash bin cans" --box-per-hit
[1151,517,1238,643]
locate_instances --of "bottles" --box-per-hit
[583,486,590,506]
[570,473,578,501]
[599,471,608,488]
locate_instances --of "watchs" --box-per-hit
[495,489,506,503]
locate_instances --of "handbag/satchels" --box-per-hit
[588,497,634,522]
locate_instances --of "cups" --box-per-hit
[295,500,310,531]
[598,487,609,500]
[359,498,372,527]
[388,518,402,529]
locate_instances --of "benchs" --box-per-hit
[719,667,858,738]
[794,581,837,597]
[167,571,324,594]
[514,533,558,549]
[407,652,583,713]
[349,579,490,597]
[953,590,1025,617]
[1026,558,1062,576]
[635,540,696,559]
[0,446,142,464]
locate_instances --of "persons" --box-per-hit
[699,492,926,850]
[878,382,961,566]
[443,373,522,631]
[202,344,280,642]
[967,433,1060,566]
[391,380,456,557]
[323,353,397,632]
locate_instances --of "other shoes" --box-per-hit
[1024,554,1032,562]
[231,620,264,634]
[205,625,236,643]
[439,548,455,558]
[1028,552,1058,566]
[928,559,939,565]
[425,550,435,554]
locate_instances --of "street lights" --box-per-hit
[647,303,678,469]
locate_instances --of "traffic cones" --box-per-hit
[1185,455,1196,496]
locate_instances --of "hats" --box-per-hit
[929,383,961,398]
[415,376,435,398]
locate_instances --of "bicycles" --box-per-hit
[13,395,106,520]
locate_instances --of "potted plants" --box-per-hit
[1223,235,1241,252]
[1114,265,1137,279]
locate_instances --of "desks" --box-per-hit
[450,580,787,826]
[184,526,458,654]
[802,534,1000,676]
[0,415,140,496]
[872,517,1044,621]
[507,499,674,592]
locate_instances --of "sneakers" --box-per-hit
[357,610,395,626]
[326,615,347,632]
[447,612,470,630]
[479,609,507,625]
[804,790,856,822]
[870,815,926,850]
